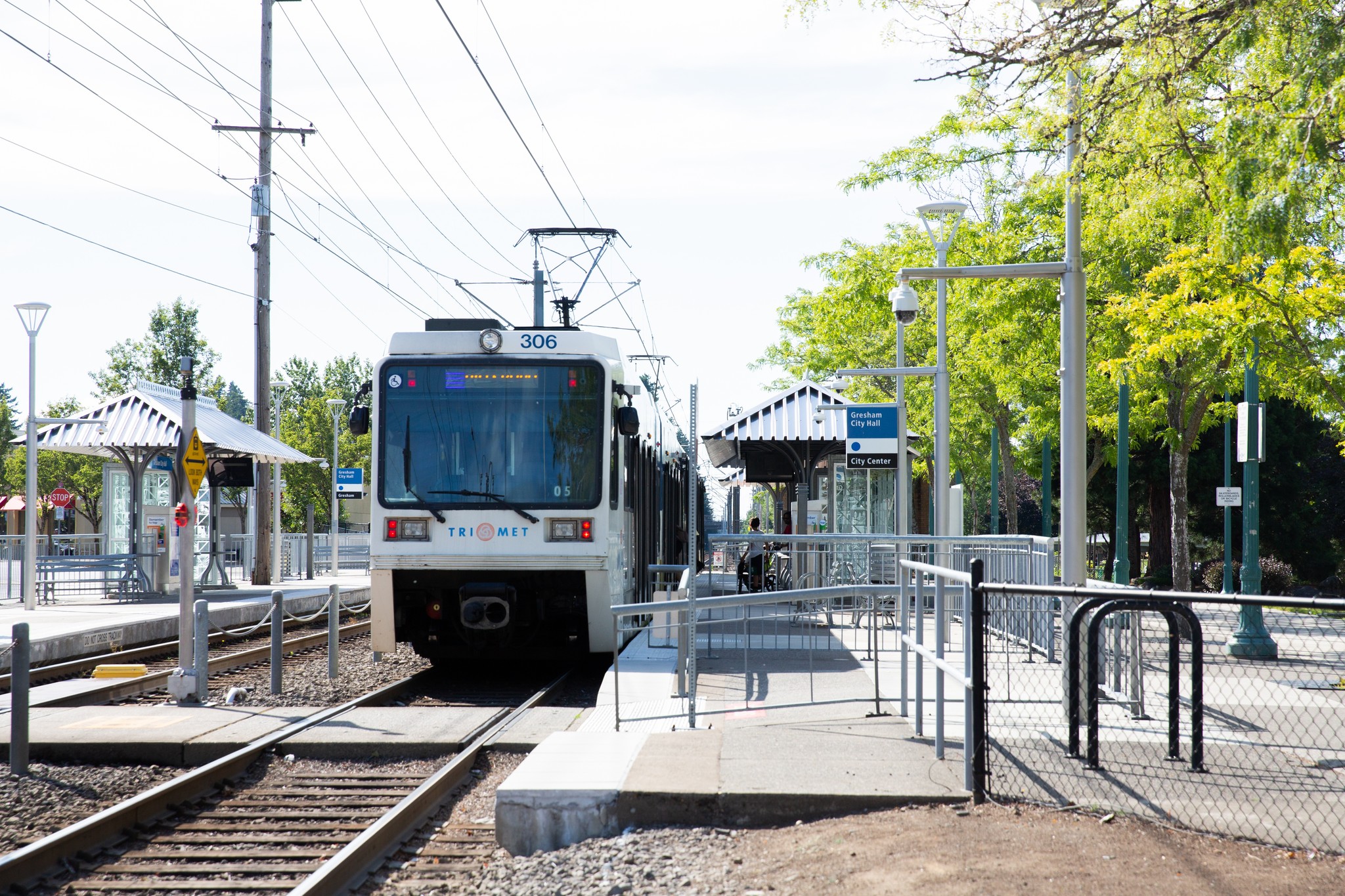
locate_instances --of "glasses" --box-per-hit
[782,517,791,520]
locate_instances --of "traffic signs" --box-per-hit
[182,427,209,499]
[336,468,363,499]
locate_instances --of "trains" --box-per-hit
[348,296,706,687]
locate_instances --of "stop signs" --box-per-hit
[51,488,70,507]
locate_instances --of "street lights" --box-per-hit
[12,302,108,611]
[325,399,347,576]
[268,381,289,584]
[890,66,1084,715]
[915,202,968,643]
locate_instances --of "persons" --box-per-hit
[773,511,794,567]
[739,517,771,594]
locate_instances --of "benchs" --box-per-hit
[846,561,899,630]
[313,545,369,576]
[35,554,134,606]
[44,554,141,605]
[789,560,844,629]
